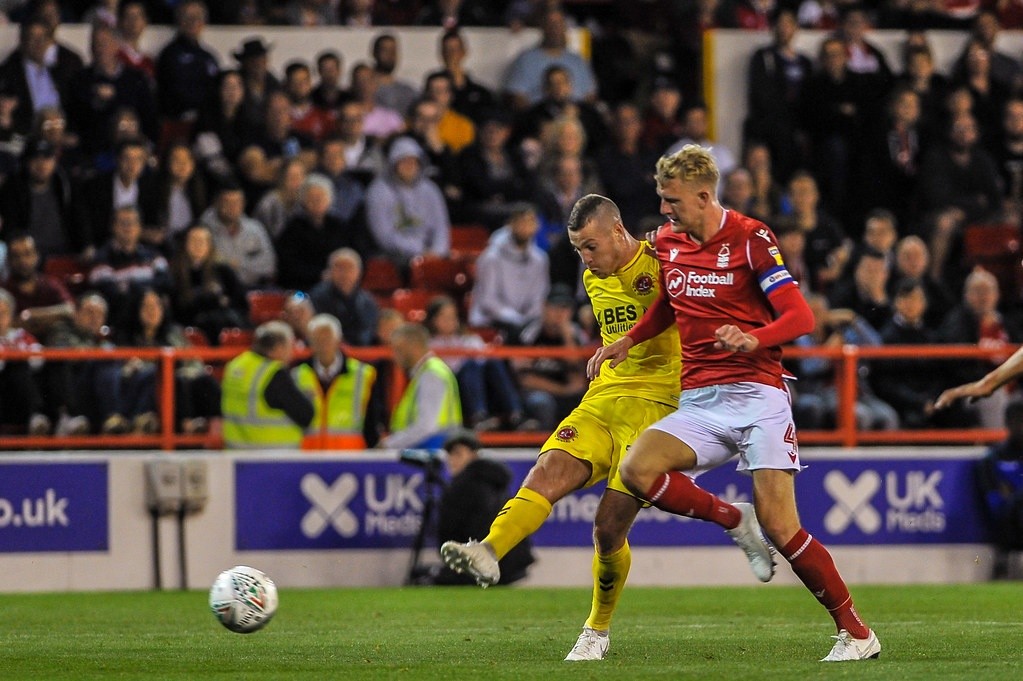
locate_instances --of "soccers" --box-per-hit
[209,565,279,634]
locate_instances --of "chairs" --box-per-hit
[360,255,405,294]
[449,225,492,254]
[964,222,1023,310]
[216,329,255,366]
[246,291,313,341]
[42,256,113,303]
[393,289,453,323]
[412,253,475,295]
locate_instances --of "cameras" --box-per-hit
[397,448,447,471]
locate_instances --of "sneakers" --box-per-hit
[724,502,778,583]
[564,630,609,661]
[821,627,881,661]
[441,537,500,590]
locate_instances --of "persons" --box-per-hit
[0,0,1023,449]
[440,194,682,660]
[960,403,1023,586]
[406,433,538,588]
[584,142,881,662]
